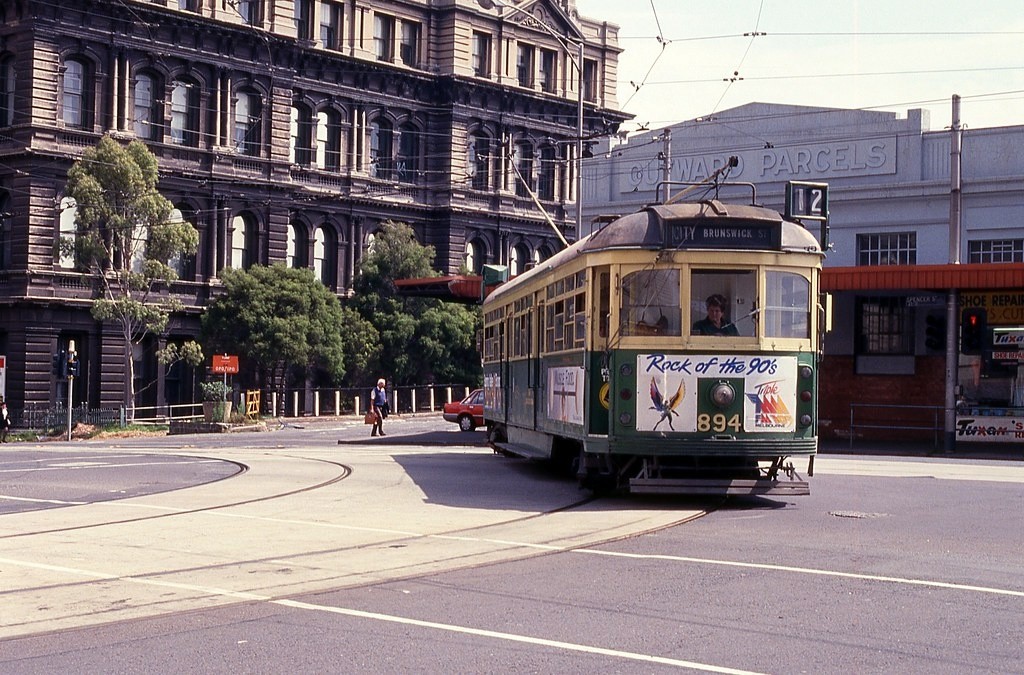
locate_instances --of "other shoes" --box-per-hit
[372,432,386,436]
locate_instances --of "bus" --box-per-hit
[476,153,833,498]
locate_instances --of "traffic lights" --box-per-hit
[961,307,988,355]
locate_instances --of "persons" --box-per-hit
[0,402,12,444]
[655,315,669,337]
[370,379,390,437]
[691,294,743,338]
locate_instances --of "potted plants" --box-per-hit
[198,382,235,423]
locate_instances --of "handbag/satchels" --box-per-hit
[365,412,379,424]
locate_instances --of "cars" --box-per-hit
[443,388,487,431]
